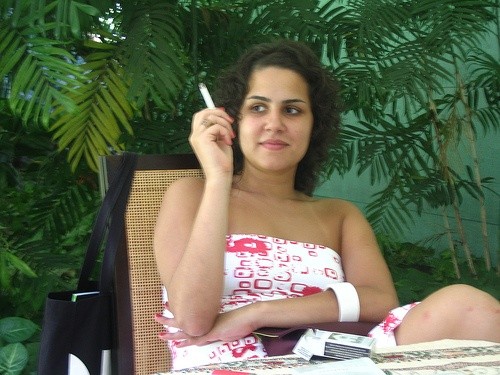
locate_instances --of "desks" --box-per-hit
[154,339,500,375]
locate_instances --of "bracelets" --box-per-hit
[326,283,360,322]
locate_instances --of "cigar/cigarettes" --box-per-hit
[197,81,216,109]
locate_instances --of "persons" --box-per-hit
[153,39,500,370]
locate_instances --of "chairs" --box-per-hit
[100,154,206,375]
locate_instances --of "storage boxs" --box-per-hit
[292,329,375,362]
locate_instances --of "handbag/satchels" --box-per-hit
[38,152,139,375]
[252,322,379,357]
